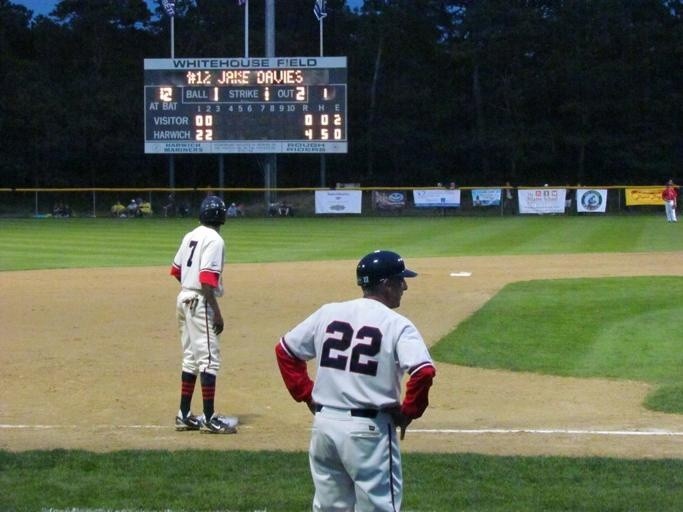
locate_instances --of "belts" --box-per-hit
[314,405,378,418]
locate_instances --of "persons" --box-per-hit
[53,201,73,219]
[169,195,239,433]
[269,201,295,217]
[662,183,677,221]
[504,183,573,215]
[112,197,153,218]
[274,249,435,512]
[437,183,457,214]
[227,201,244,217]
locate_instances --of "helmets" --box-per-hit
[199,195,226,224]
[357,251,417,287]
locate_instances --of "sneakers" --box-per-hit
[175,410,237,434]
